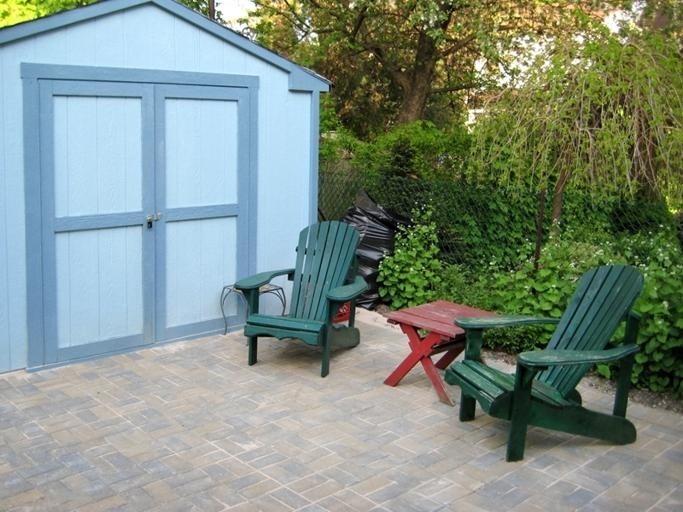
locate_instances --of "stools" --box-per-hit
[219,284,287,335]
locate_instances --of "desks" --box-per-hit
[383,298,495,406]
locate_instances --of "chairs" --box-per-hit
[444,265,645,461]
[234,219,368,378]
